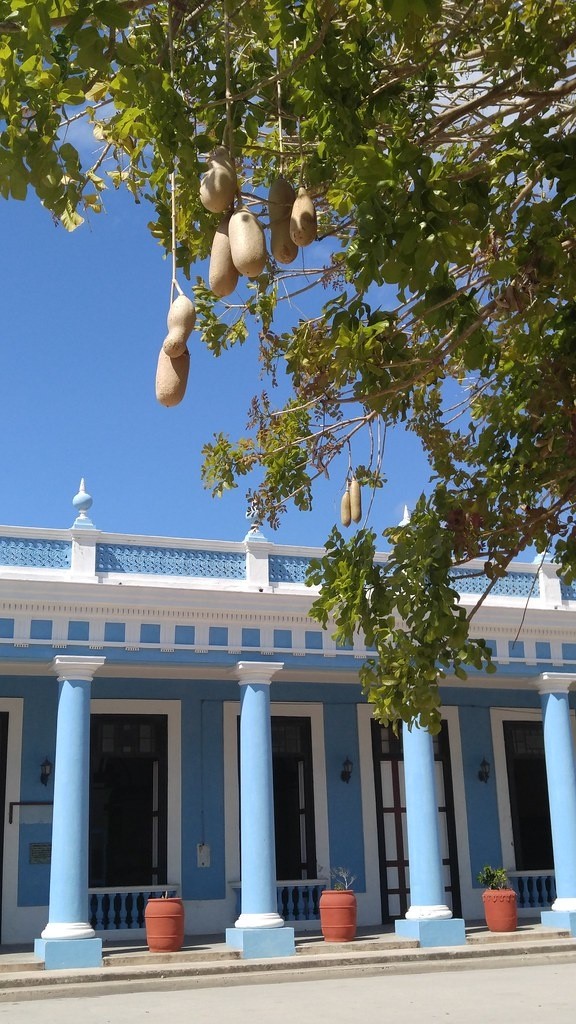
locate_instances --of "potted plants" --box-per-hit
[477,865,517,933]
[144,891,184,953]
[319,866,358,942]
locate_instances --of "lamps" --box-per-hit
[478,757,491,782]
[340,756,353,784]
[40,755,52,786]
